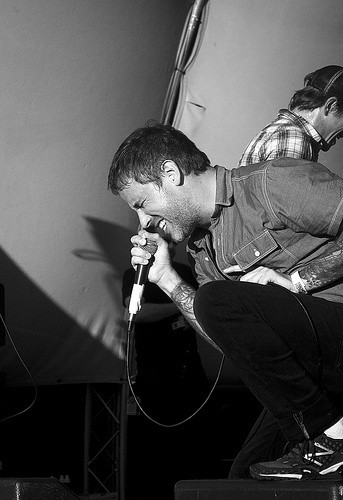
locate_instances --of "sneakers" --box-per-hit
[249,434,343,481]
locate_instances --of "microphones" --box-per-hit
[126,234,157,331]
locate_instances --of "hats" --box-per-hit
[306,64,342,103]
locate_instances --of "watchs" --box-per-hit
[290,270,309,295]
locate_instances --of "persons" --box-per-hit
[105,119,343,481]
[238,65,343,167]
